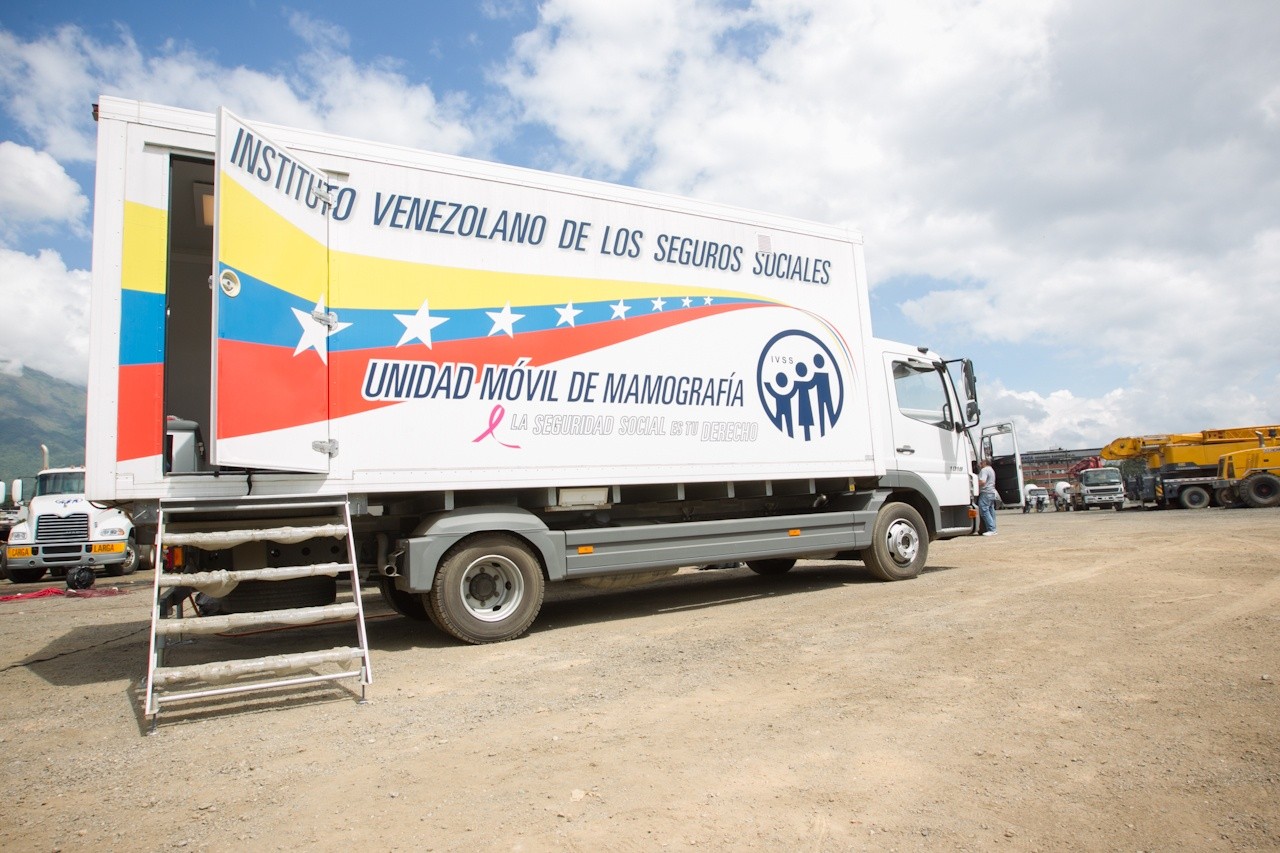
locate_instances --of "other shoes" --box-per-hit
[982,530,998,537]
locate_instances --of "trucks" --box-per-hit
[1026,481,1075,508]
[1,467,157,584]
[1063,467,1128,511]
[86,92,1025,646]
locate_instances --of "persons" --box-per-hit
[1053,491,1059,512]
[978,458,997,536]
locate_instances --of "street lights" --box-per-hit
[1059,448,1070,482]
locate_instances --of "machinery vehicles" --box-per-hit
[1100,425,1280,509]
